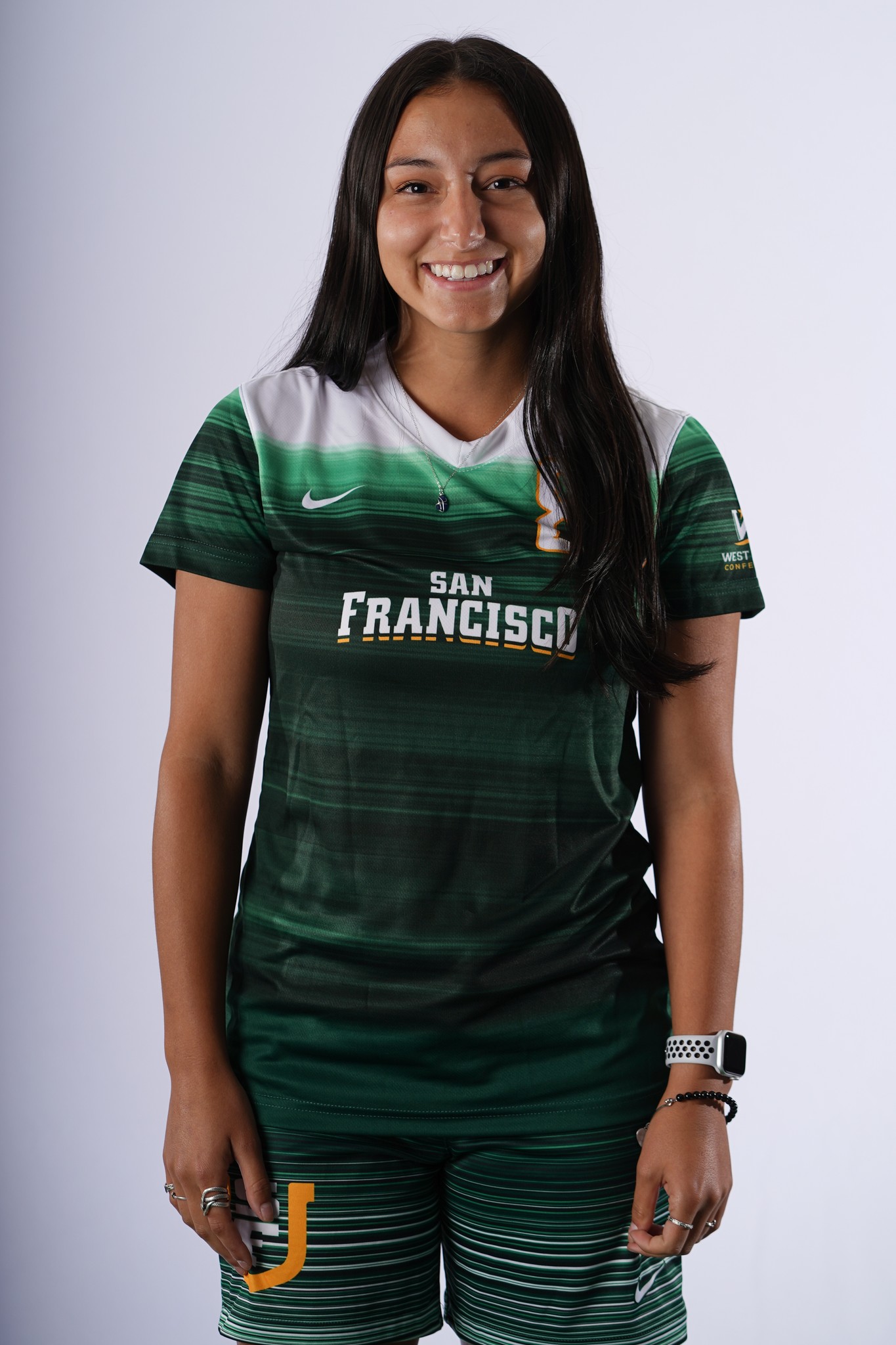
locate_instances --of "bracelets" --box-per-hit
[636,1091,737,1147]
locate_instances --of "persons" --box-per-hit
[136,33,766,1345]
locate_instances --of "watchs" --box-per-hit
[664,1031,746,1081]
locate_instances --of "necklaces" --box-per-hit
[392,356,538,513]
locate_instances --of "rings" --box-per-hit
[705,1220,718,1228]
[200,1187,230,1216]
[667,1215,694,1231]
[164,1182,175,1193]
[172,1192,187,1201]
